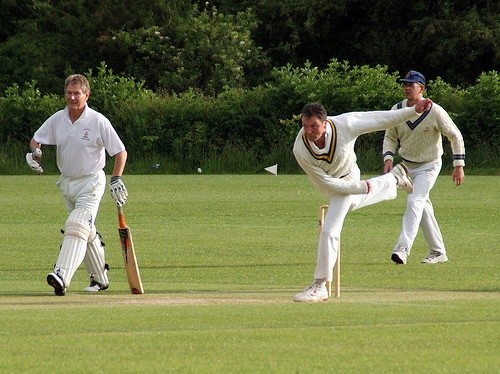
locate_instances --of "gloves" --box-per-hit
[110,176,128,207]
[26,148,43,172]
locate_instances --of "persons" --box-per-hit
[382,71,465,265]
[29,74,129,295]
[292,97,433,302]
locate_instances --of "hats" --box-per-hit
[400,70,425,85]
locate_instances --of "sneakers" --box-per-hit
[293,282,328,302]
[421,251,448,263]
[391,247,406,264]
[391,162,413,194]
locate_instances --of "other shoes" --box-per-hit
[83,285,108,292]
[47,273,65,296]
